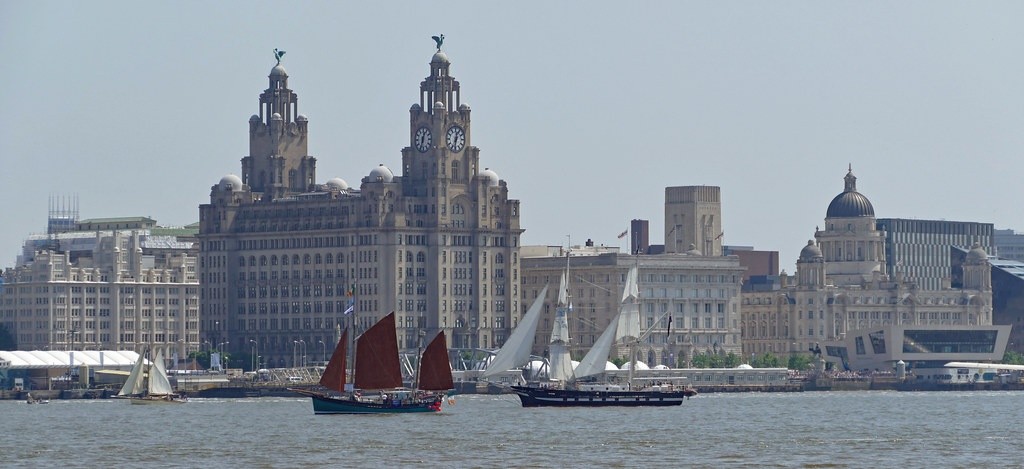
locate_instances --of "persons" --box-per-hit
[346,388,442,405]
[540,379,685,392]
[795,368,1024,383]
[11,383,188,402]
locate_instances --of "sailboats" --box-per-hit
[116,328,188,404]
[474,234,686,408]
[286,279,457,416]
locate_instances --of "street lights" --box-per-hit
[249,339,259,371]
[300,339,307,367]
[318,340,326,371]
[293,341,302,368]
